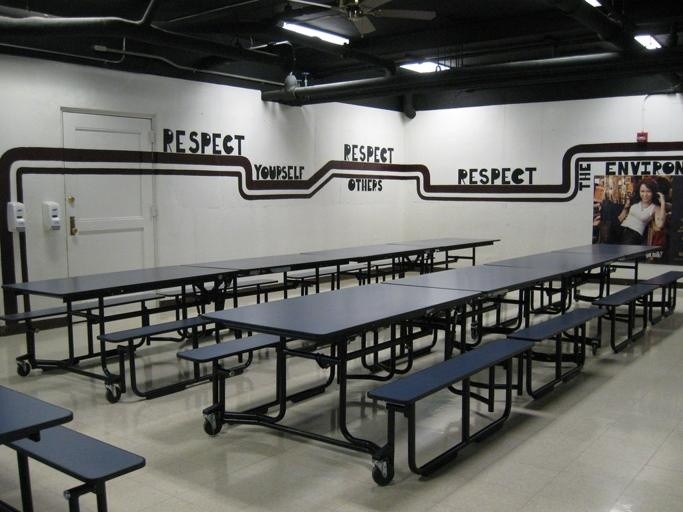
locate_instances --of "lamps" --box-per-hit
[273,17,350,46]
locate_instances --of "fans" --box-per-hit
[287,0,438,39]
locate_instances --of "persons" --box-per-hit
[593,176,683,258]
